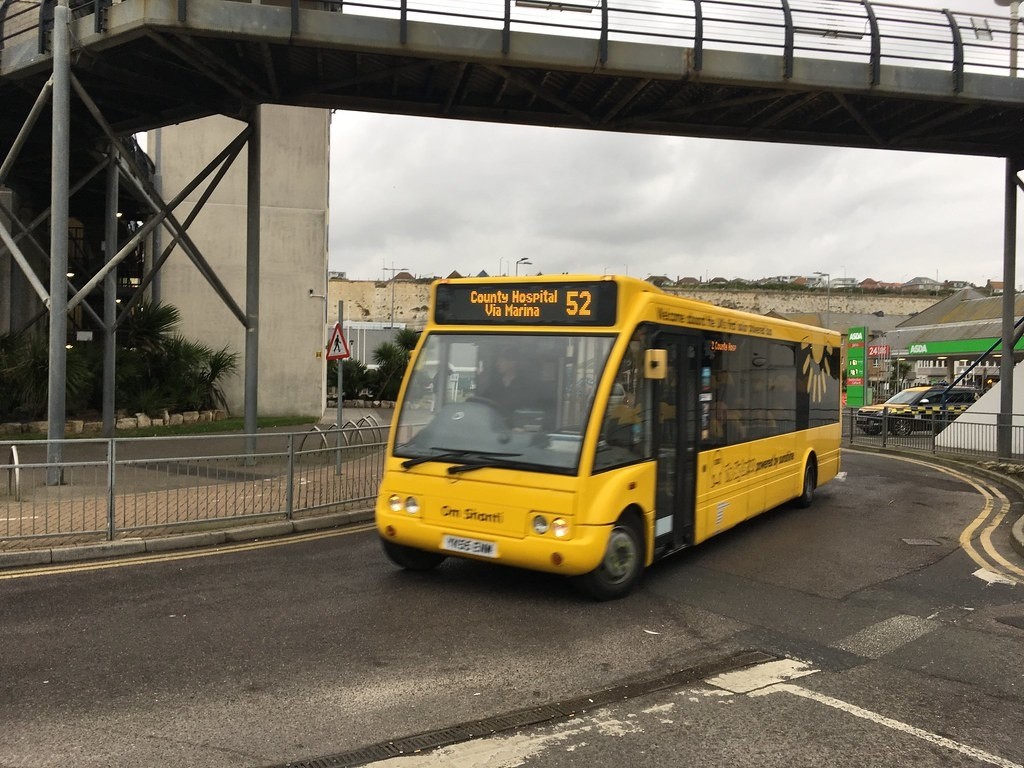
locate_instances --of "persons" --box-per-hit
[484,344,556,432]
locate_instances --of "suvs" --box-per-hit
[853,386,982,437]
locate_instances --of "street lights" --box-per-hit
[498,257,503,277]
[515,256,532,276]
[813,272,832,329]
[381,267,410,329]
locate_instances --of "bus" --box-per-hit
[367,272,843,599]
